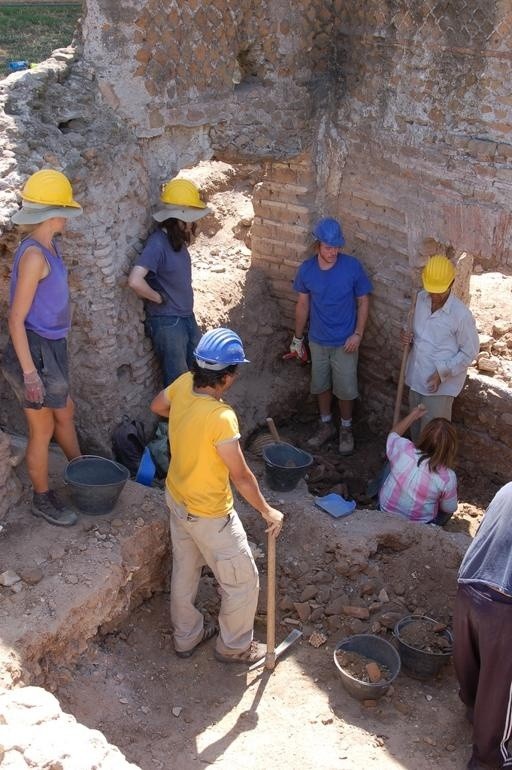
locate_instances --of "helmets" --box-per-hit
[310,218,346,266]
[193,327,252,371]
[21,170,83,209]
[420,255,456,296]
[160,178,206,208]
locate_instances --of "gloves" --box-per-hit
[292,336,304,365]
[23,369,46,406]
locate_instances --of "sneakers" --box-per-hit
[31,488,78,526]
[338,423,356,455]
[170,621,219,658]
[306,419,336,448]
[214,640,271,664]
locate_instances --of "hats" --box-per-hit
[153,203,211,224]
[9,199,81,225]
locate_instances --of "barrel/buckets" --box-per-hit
[393,613,455,682]
[262,439,314,493]
[332,633,402,702]
[65,454,131,516]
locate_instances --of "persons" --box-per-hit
[1,169,85,528]
[399,255,479,452]
[451,479,512,769]
[151,327,285,662]
[127,179,214,387]
[375,404,460,527]
[287,218,373,457]
